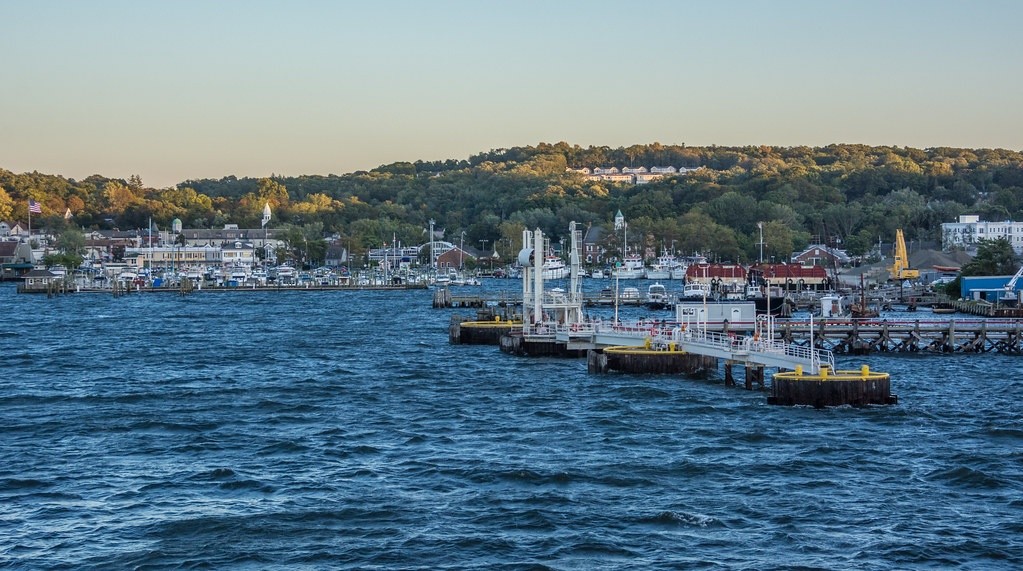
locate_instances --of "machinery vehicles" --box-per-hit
[886,229,920,283]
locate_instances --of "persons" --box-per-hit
[711,276,723,287]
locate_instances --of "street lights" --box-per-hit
[479,240,489,251]
[460,231,467,268]
[761,273,775,350]
[698,264,710,341]
[612,265,626,331]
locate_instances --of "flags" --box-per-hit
[29,199,42,213]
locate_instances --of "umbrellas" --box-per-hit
[895,230,909,299]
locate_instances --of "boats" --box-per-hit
[646,283,669,305]
[620,287,641,304]
[678,222,926,326]
[599,289,615,305]
[545,287,566,302]
[485,301,498,309]
[932,305,957,314]
[476,212,707,280]
[49,217,481,288]
